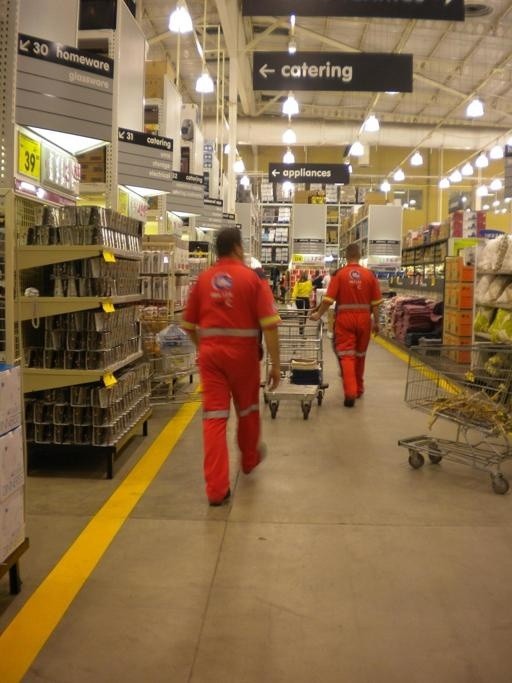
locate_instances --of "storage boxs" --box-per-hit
[442,208,486,363]
[0,424,24,502]
[0,486,25,564]
[0,365,21,437]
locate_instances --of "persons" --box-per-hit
[322,270,330,289]
[289,273,313,336]
[310,243,385,407]
[179,226,283,507]
[312,271,323,290]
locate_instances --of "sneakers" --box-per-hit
[244,442,267,474]
[210,488,231,505]
[344,399,355,406]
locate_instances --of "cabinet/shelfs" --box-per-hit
[323,201,447,367]
[0,187,30,598]
[469,243,511,397]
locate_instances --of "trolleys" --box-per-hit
[262,311,328,414]
[397,338,512,499]
[133,320,201,399]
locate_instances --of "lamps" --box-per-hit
[282,41,300,200]
[167,0,252,191]
[380,97,512,214]
[333,114,379,186]
